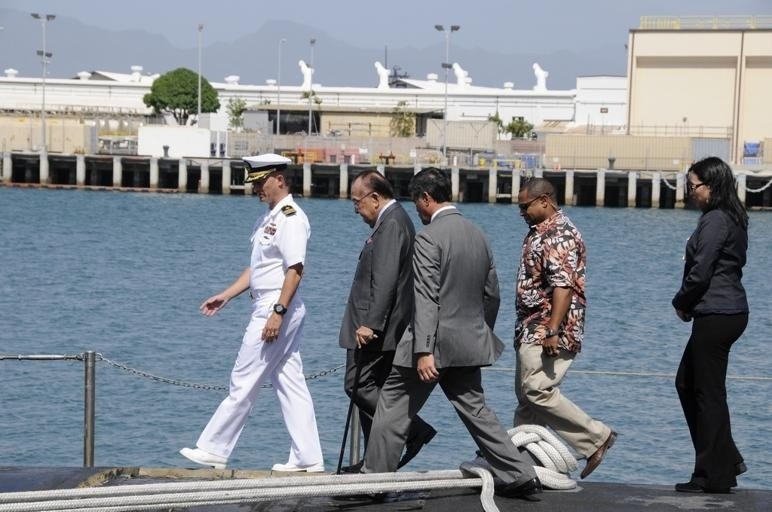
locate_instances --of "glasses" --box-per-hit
[519,192,550,210]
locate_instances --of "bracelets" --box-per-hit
[544,326,557,338]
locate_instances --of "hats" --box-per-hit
[241,153,293,183]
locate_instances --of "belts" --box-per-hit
[248,286,266,301]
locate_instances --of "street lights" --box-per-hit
[31,9,55,145]
[307,37,317,137]
[433,24,461,162]
[34,49,53,79]
[441,61,453,121]
[195,22,204,128]
[276,38,287,134]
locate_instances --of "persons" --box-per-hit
[359,166,543,499]
[178,152,327,473]
[337,169,438,473]
[513,177,619,480]
[670,155,750,494]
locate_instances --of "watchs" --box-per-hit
[272,303,287,316]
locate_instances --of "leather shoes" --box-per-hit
[396,424,437,469]
[341,459,364,472]
[675,482,730,493]
[734,462,747,476]
[333,493,384,500]
[496,476,543,495]
[179,447,227,470]
[270,460,326,473]
[580,428,618,479]
